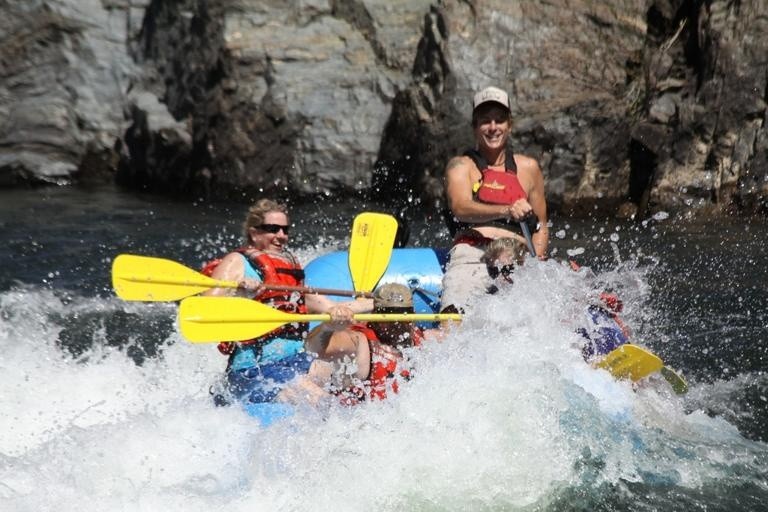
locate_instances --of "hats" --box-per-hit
[374,285,412,308]
[472,87,509,109]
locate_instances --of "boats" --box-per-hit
[223,248,631,436]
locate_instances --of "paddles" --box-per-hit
[179,295,465,343]
[111,255,378,302]
[592,344,664,386]
[348,211,399,298]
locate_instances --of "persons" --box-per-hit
[484,238,629,380]
[424,87,549,346]
[304,284,429,409]
[195,200,357,431]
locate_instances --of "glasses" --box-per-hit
[254,224,291,234]
[487,263,527,278]
